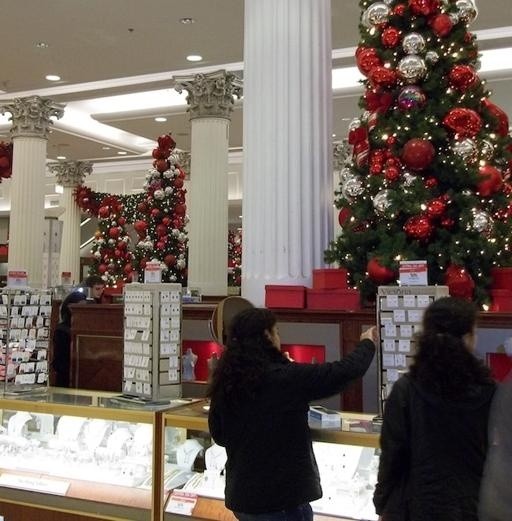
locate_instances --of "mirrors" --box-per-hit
[207,295,257,352]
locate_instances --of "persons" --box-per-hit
[206,308,377,517]
[51,291,86,388]
[371,295,498,518]
[85,274,105,304]
[475,372,512,521]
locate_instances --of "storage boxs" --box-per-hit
[263,268,360,315]
[483,260,512,311]
